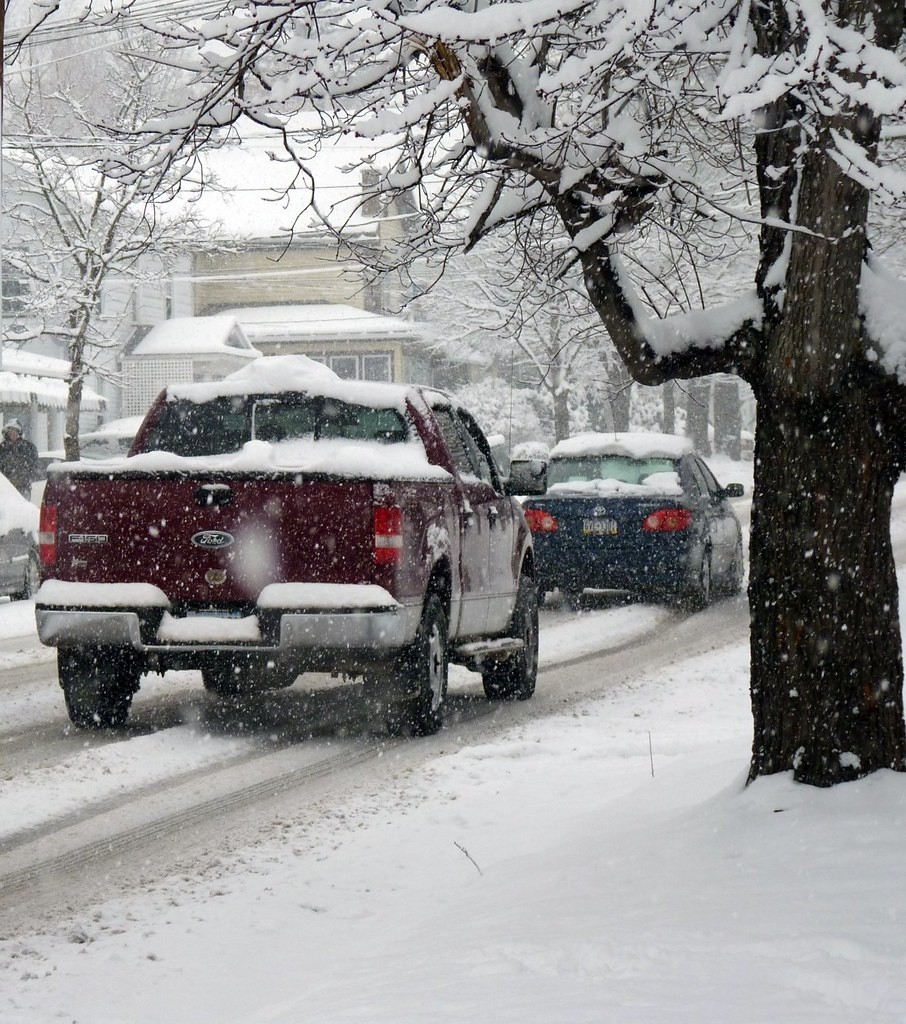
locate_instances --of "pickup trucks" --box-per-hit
[29,382,547,732]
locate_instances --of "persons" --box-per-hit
[0,418,38,502]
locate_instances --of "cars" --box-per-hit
[28,451,95,508]
[0,471,44,600]
[521,431,743,610]
